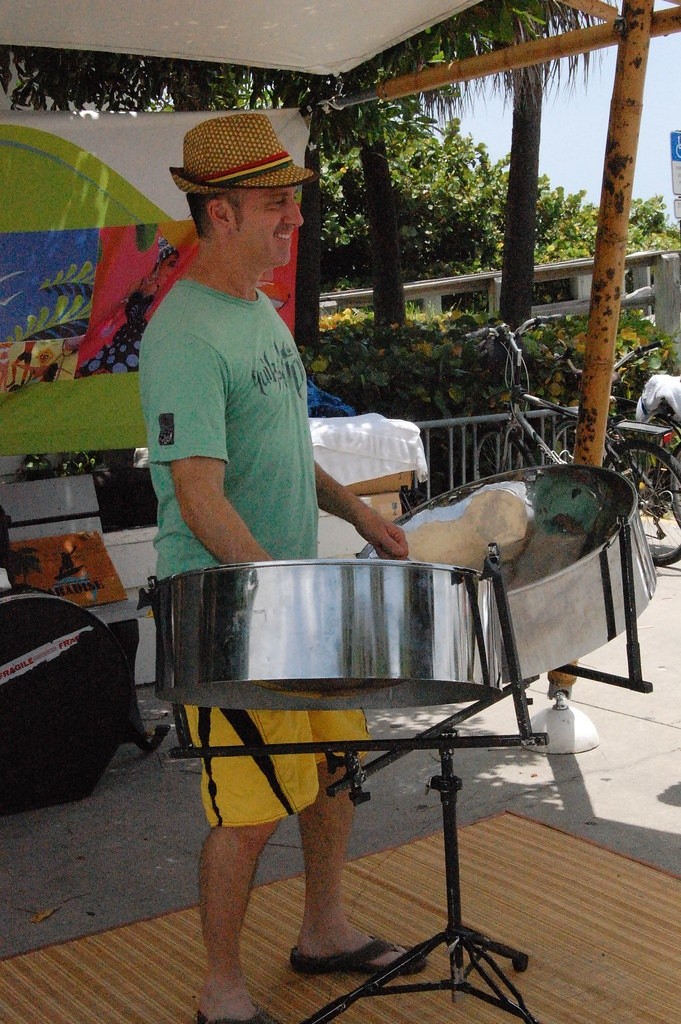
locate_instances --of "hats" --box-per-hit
[169,114,320,193]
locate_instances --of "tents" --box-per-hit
[0,0,681,754]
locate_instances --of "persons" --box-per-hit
[139,114,409,1024]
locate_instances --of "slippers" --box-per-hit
[290,935,426,975]
[197,1000,281,1024]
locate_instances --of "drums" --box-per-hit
[356,464,657,684]
[0,588,132,818]
[155,558,503,711]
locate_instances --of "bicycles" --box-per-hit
[459,314,681,567]
